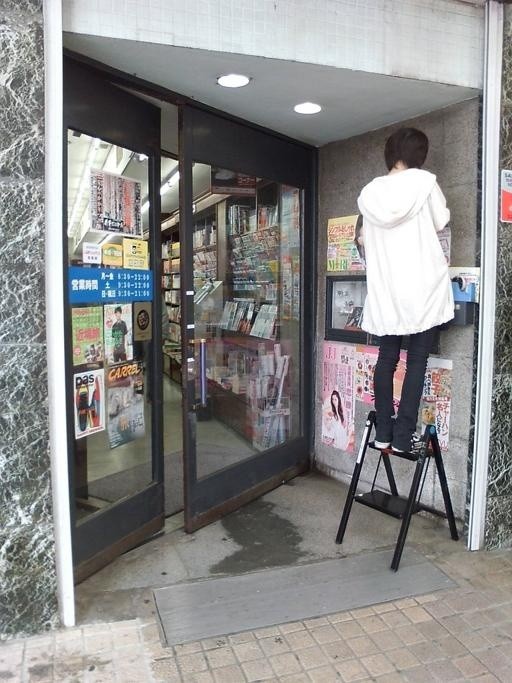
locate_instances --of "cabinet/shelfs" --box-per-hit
[154,175,292,453]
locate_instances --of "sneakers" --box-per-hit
[391,435,420,453]
[373,439,392,449]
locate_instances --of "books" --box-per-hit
[160,201,291,451]
[343,305,364,331]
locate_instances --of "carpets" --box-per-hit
[151,544,460,650]
[80,441,277,519]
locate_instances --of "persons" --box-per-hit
[324,390,352,450]
[131,246,136,254]
[347,310,359,328]
[117,416,132,442]
[111,307,128,362]
[356,126,452,453]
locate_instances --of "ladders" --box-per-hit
[336,411,460,572]
